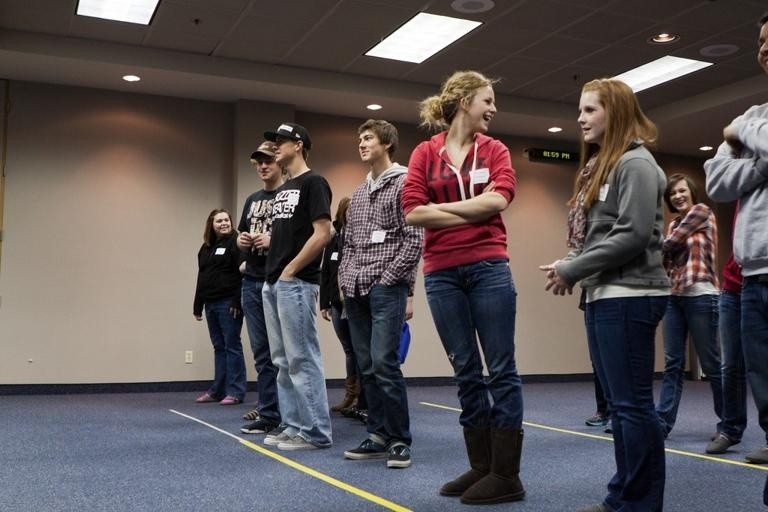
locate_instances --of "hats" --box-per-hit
[251,141,276,159]
[264,122,311,149]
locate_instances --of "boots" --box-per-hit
[332,378,368,424]
[440,426,526,505]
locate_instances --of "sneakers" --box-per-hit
[344,438,390,459]
[706,432,741,452]
[386,445,411,468]
[220,395,239,405]
[585,413,612,432]
[195,393,215,401]
[241,408,318,451]
[745,446,768,463]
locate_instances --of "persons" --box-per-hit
[400,70,524,504]
